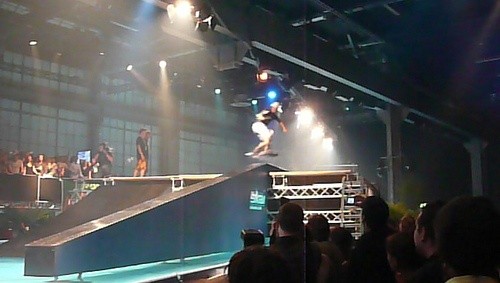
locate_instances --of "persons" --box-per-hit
[92,143,114,178]
[251,102,288,154]
[0,150,92,178]
[271,203,321,283]
[348,196,396,283]
[386,199,461,283]
[227,203,354,283]
[435,196,500,283]
[134,129,152,177]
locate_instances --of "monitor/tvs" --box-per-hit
[76,149,92,165]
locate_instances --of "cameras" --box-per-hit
[345,197,356,205]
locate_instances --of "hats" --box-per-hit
[270,102,281,108]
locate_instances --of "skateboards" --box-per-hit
[244,152,279,159]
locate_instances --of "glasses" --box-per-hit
[420,203,431,214]
